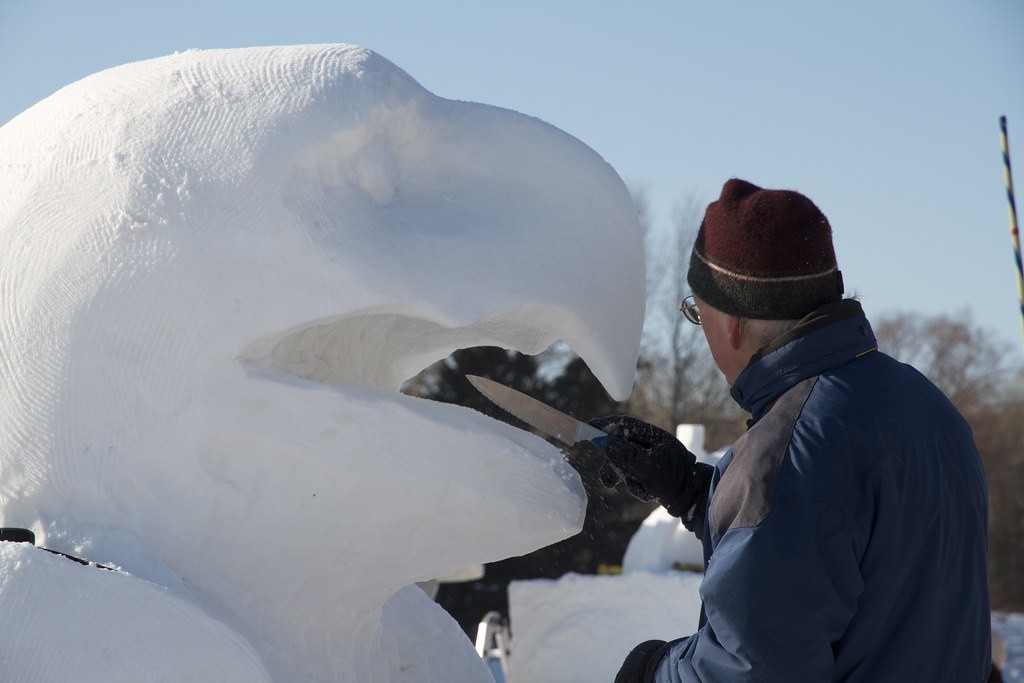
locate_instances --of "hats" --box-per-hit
[687,178,844,320]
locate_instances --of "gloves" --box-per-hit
[614,635,687,682]
[585,414,699,517]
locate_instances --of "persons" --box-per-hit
[583,176,996,683]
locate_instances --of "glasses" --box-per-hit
[679,296,704,326]
